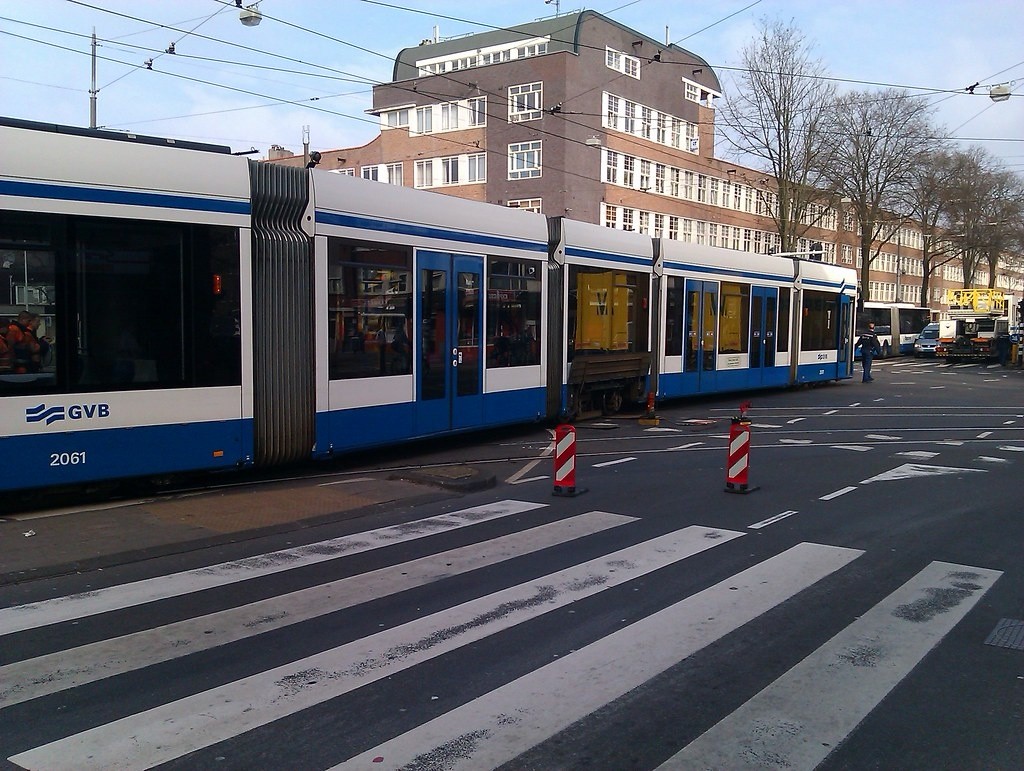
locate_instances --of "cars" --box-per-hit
[913,323,940,358]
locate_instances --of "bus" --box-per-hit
[852,300,932,360]
[0,118,865,497]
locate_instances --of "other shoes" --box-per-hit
[870,378,874,381]
[862,380,872,383]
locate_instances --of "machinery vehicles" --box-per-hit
[936,289,1023,367]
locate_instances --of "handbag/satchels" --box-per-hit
[391,341,402,352]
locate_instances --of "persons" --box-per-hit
[394,325,408,345]
[855,324,880,383]
[375,325,386,363]
[0,310,46,374]
